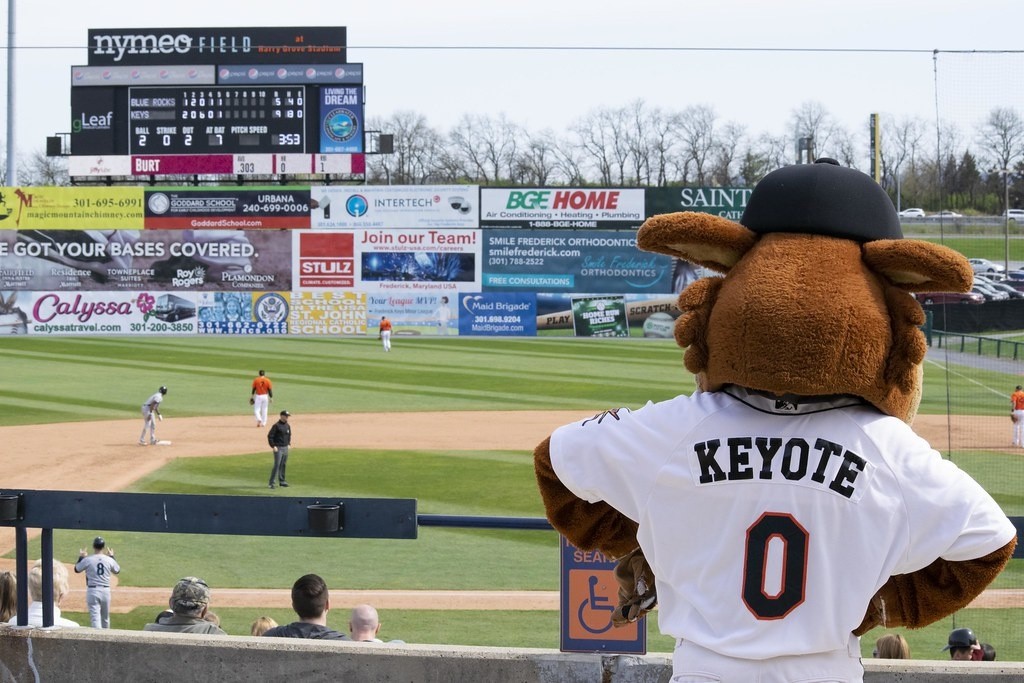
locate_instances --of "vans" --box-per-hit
[1002,208,1024,226]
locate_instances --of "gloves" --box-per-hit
[147,413,152,421]
[158,414,163,421]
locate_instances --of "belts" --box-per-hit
[88,585,109,588]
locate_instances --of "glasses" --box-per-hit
[873,650,879,657]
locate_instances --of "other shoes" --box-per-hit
[269,483,275,488]
[139,441,148,446]
[279,483,289,487]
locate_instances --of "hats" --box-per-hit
[173,577,210,610]
[259,370,264,375]
[1016,385,1022,390]
[281,411,291,416]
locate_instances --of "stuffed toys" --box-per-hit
[533,157,1018,683]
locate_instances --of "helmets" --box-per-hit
[94,537,105,548]
[160,386,168,394]
[942,627,981,651]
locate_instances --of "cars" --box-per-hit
[969,258,1005,274]
[897,208,926,218]
[930,210,962,220]
[917,287,986,307]
[971,267,1024,302]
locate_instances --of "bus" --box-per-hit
[155,293,196,322]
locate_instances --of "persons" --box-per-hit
[980,643,996,661]
[872,633,911,659]
[379,316,392,352]
[139,386,167,445]
[251,616,278,636]
[349,603,383,643]
[143,577,228,635]
[0,571,18,623]
[268,410,292,489]
[940,628,984,663]
[1010,385,1024,447]
[249,370,272,427]
[74,536,120,629]
[8,558,80,627]
[262,574,352,642]
[432,296,450,336]
